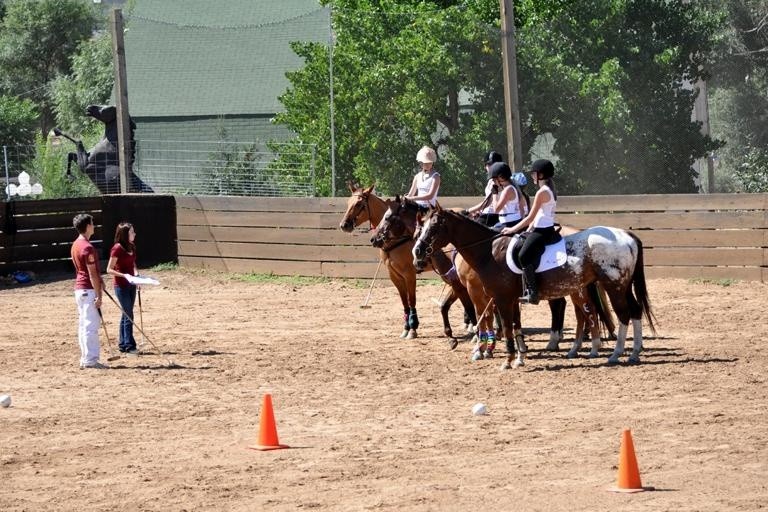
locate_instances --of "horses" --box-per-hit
[412,210,616,360]
[492,280,617,341]
[412,199,660,370]
[54,104,156,195]
[370,193,567,352]
[339,184,470,340]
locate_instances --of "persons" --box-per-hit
[486,162,520,231]
[71,213,110,368]
[451,150,502,226]
[106,222,139,352]
[501,159,557,304]
[511,172,530,216]
[404,145,441,213]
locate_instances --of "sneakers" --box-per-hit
[117,349,141,356]
[80,362,106,369]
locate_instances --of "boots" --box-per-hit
[518,264,539,305]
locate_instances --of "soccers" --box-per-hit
[1,394,12,407]
[472,403,488,416]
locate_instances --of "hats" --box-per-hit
[510,172,527,187]
[416,146,437,164]
[487,161,512,179]
[527,159,555,179]
[482,150,502,164]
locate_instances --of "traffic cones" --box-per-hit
[248,393,290,450]
[607,431,656,495]
